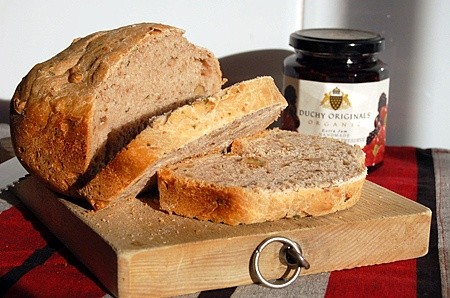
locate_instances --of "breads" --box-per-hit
[8,22,224,199]
[156,127,369,226]
[78,75,288,210]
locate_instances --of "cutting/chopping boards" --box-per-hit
[0,135,432,298]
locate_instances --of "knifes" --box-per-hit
[0,156,30,195]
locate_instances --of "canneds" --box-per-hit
[279,28,389,171]
